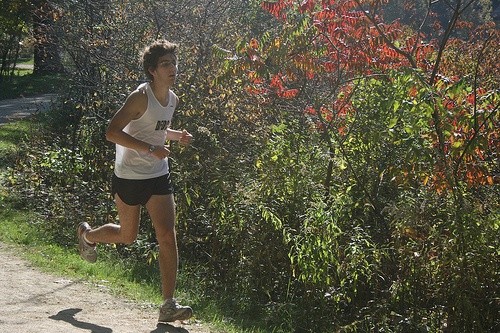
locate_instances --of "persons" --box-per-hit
[75,39,194,323]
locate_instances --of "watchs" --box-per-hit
[149,144,157,154]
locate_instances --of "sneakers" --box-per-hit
[158,297,192,322]
[76,221,98,263]
